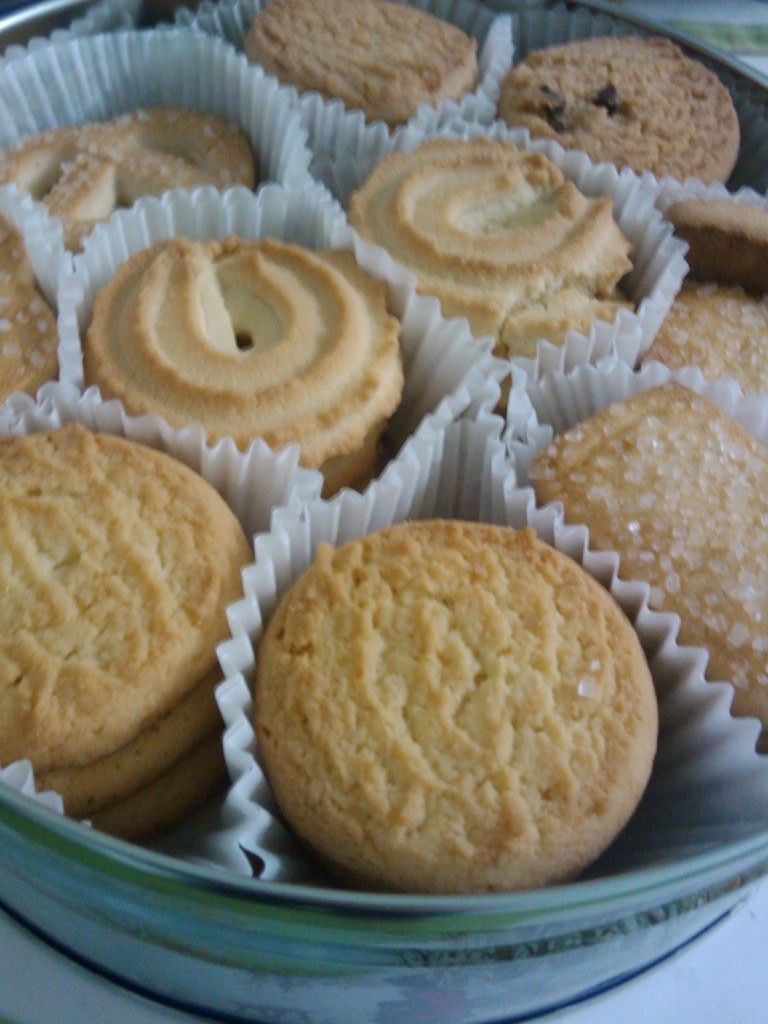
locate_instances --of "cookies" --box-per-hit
[0,0,767,887]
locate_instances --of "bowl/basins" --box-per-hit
[0,0,768,1024]
